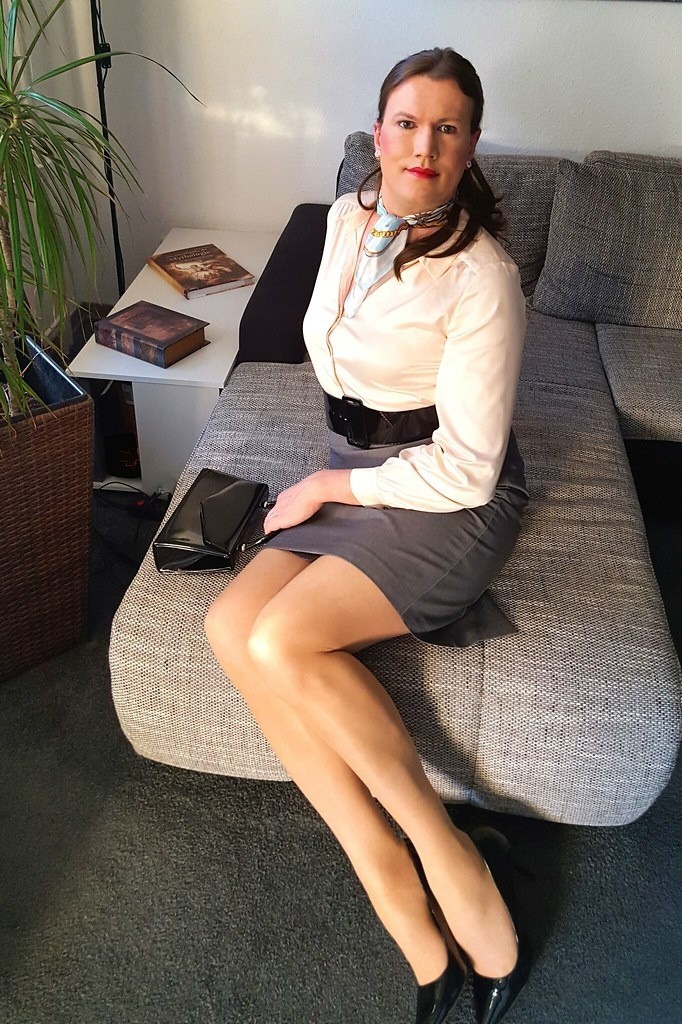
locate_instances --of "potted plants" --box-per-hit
[0,0,199,685]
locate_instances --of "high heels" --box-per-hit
[403,837,465,1024]
[463,829,531,1024]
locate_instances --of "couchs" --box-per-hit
[107,129,682,825]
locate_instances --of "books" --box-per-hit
[95,301,210,369]
[147,243,255,300]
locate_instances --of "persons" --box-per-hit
[205,48,534,1024]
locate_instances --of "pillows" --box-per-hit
[522,159,681,330]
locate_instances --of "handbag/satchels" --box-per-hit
[151,467,286,576]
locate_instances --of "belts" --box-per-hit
[321,388,438,450]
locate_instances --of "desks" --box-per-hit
[62,228,285,498]
